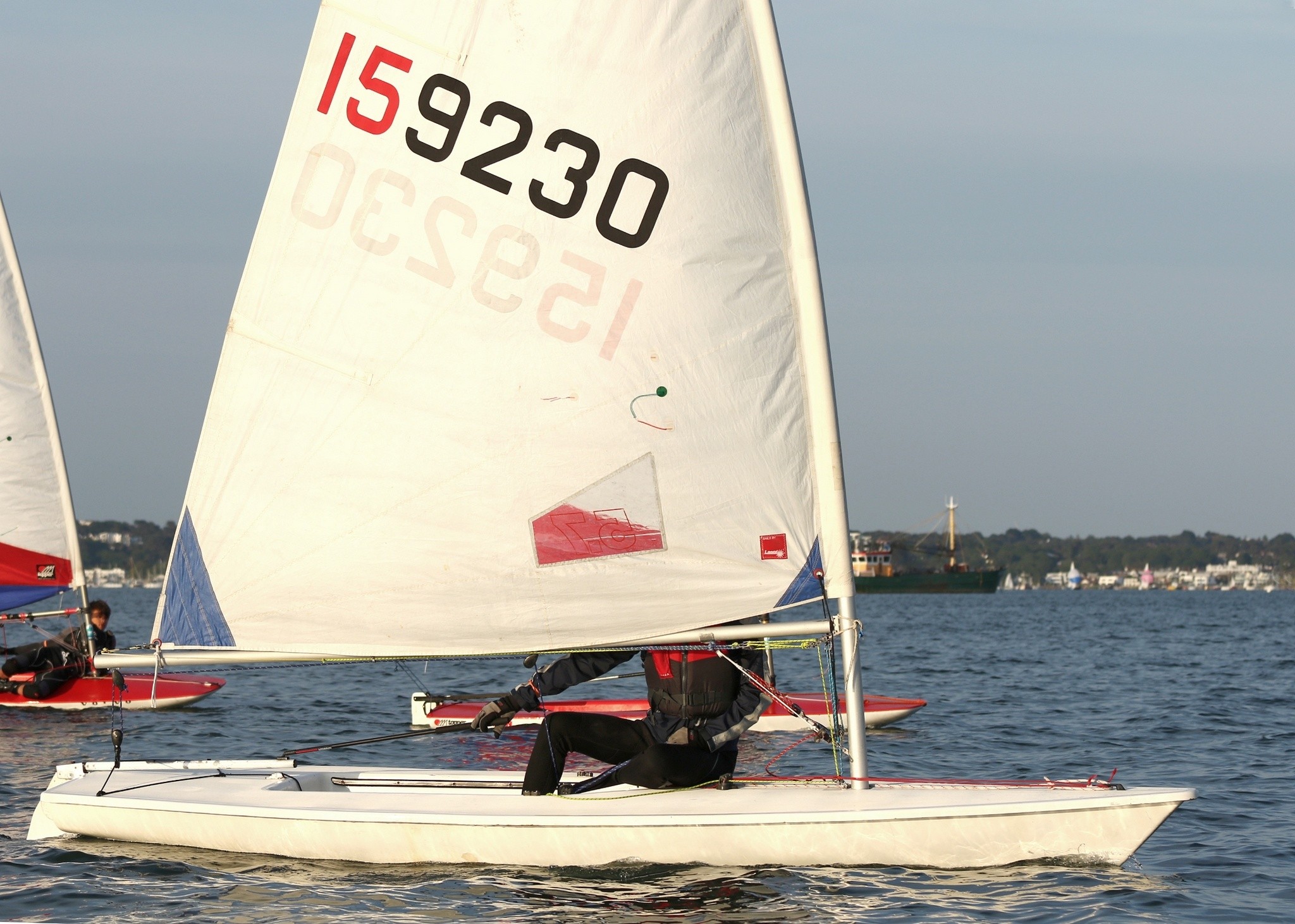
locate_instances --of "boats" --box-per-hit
[996,555,1281,594]
[87,571,165,590]
[408,682,930,733]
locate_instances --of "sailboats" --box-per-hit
[25,0,1207,869]
[1,194,226,713]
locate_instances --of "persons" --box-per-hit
[0,600,116,701]
[471,618,772,797]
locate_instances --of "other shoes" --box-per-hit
[0,678,23,695]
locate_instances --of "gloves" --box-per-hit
[664,726,699,744]
[469,696,519,739]
